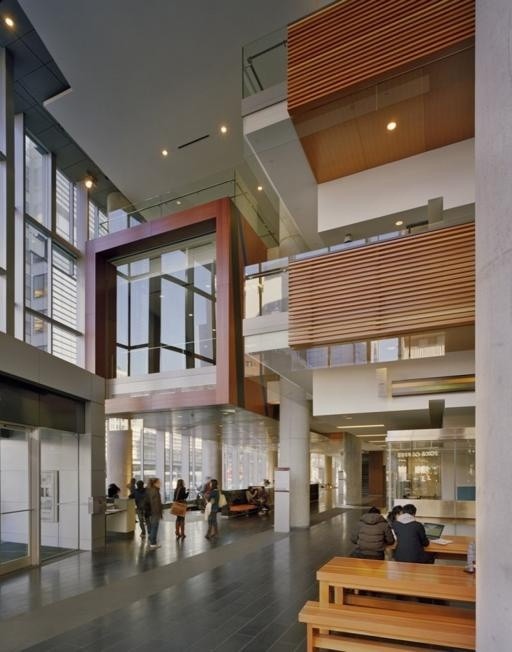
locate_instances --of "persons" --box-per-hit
[347,507,395,597]
[245,480,270,516]
[385,506,402,562]
[173,479,190,539]
[108,483,120,499]
[204,479,219,539]
[393,504,432,605]
[126,478,164,550]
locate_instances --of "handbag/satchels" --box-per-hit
[218,491,227,508]
[169,488,187,518]
[140,494,151,518]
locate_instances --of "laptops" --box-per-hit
[423,523,444,540]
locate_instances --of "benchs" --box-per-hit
[222,488,261,519]
[298,600,474,651]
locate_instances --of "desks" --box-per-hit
[315,534,476,651]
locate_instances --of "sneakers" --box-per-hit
[261,507,268,510]
[258,511,265,515]
[150,544,161,548]
